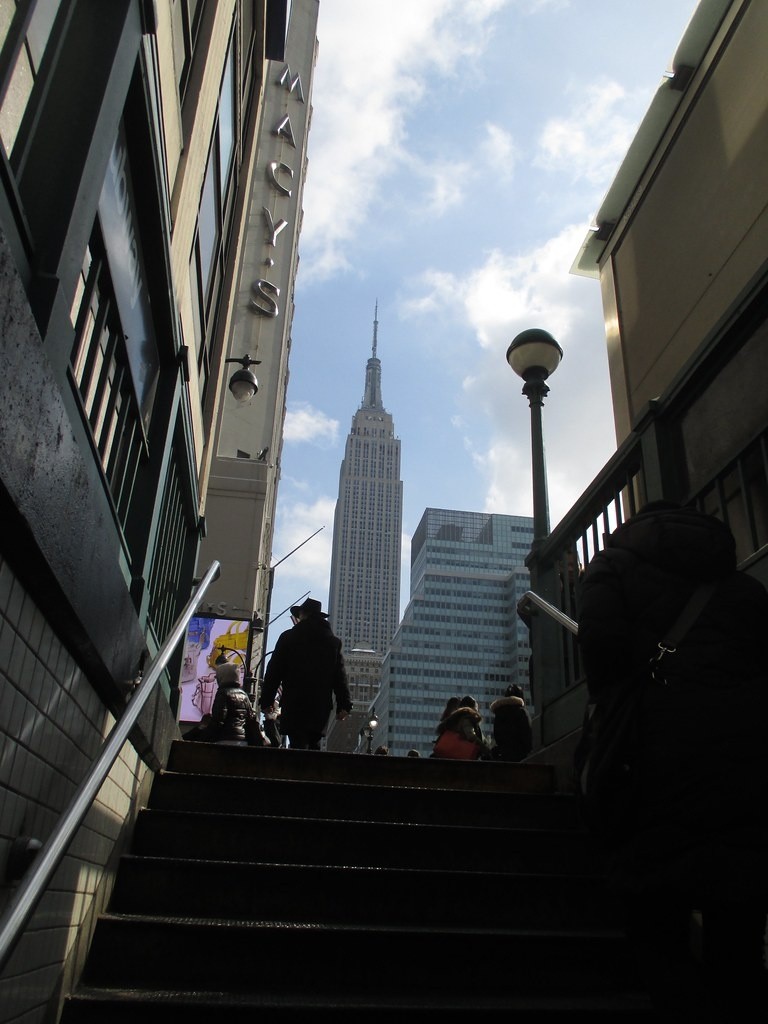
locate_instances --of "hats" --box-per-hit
[215,662,239,684]
[290,598,330,619]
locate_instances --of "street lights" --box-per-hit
[506,328,562,548]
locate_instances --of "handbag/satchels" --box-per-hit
[434,719,480,760]
[243,703,262,742]
[573,676,647,790]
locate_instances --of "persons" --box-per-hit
[490,685,532,762]
[201,664,254,744]
[262,598,354,750]
[575,500,768,1024]
[437,696,496,760]
